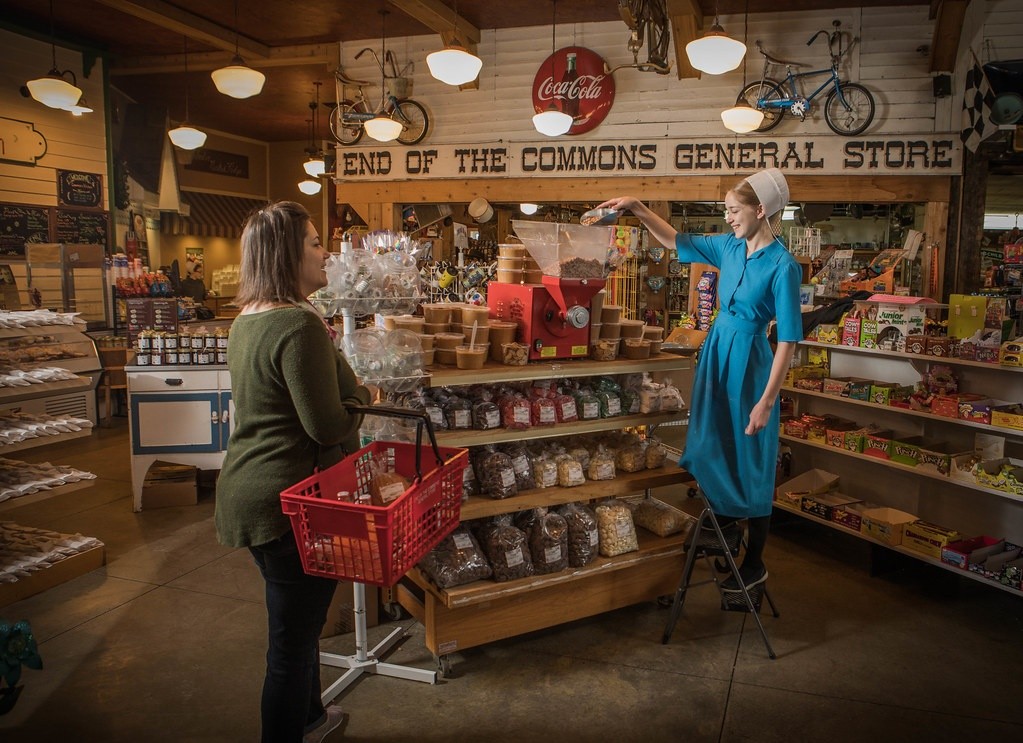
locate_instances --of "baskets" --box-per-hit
[385,76,408,97]
[279,407,473,588]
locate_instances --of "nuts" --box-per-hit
[529,440,691,557]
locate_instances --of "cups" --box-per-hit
[482,262,497,288]
[433,264,459,289]
[461,261,486,289]
[465,287,487,306]
[445,293,460,302]
[110,253,164,329]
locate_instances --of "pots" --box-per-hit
[468,196,494,224]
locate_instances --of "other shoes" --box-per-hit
[303,705,344,743]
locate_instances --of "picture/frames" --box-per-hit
[57,167,104,210]
[0,200,54,260]
[52,206,113,256]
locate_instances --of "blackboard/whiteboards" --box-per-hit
[0,200,112,259]
[56,168,104,211]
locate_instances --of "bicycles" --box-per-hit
[603,0,674,75]
[328,47,429,146]
[735,29,875,137]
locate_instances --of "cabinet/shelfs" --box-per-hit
[0,331,102,456]
[773,339,1023,596]
[382,352,695,655]
[185,247,205,280]
[125,357,237,512]
[0,316,106,607]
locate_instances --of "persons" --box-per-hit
[993,266,1004,286]
[213,201,379,743]
[596,166,804,593]
[181,262,206,304]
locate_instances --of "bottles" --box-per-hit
[363,324,389,343]
[359,494,385,580]
[382,328,422,391]
[380,251,421,315]
[357,400,432,474]
[923,358,960,395]
[339,330,383,390]
[337,491,360,577]
[993,266,1004,286]
[335,249,380,314]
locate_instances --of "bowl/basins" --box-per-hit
[590,289,663,361]
[494,243,546,284]
[402,206,418,226]
[383,303,530,369]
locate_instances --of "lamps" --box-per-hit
[721,0,765,134]
[685,0,746,73]
[297,80,327,196]
[212,3,266,101]
[364,1,404,141]
[426,0,482,85]
[28,0,82,111]
[531,0,575,138]
[167,34,208,150]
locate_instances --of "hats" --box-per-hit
[743,168,790,218]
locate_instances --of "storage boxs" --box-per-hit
[779,295,1023,588]
[140,459,199,508]
[319,578,379,639]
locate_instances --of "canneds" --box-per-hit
[137,330,232,366]
[92,336,129,347]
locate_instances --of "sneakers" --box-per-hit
[700,510,748,530]
[721,561,769,592]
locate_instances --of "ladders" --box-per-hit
[660,484,780,660]
